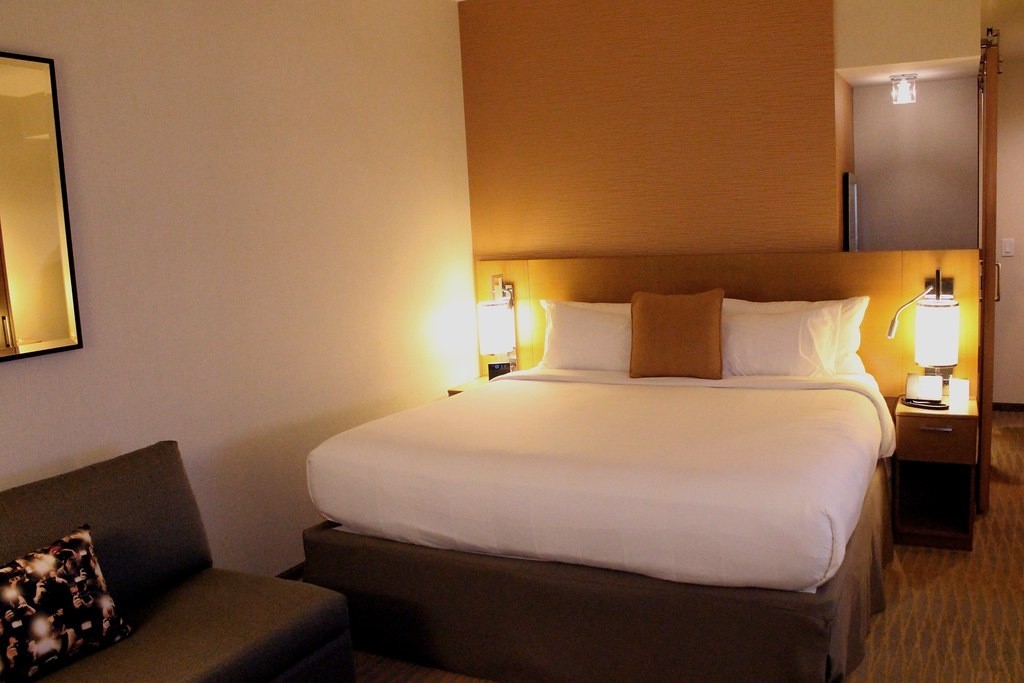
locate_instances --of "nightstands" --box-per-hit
[447,374,491,396]
[896,400,979,551]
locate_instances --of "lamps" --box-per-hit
[916,297,961,405]
[476,298,517,354]
[891,73,918,106]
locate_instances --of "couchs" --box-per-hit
[0,440,355,683]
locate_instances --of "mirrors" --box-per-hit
[0,50,84,362]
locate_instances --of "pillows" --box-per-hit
[629,288,722,382]
[721,295,874,376]
[537,298,631,371]
[0,525,131,683]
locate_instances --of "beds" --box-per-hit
[303,372,898,683]
